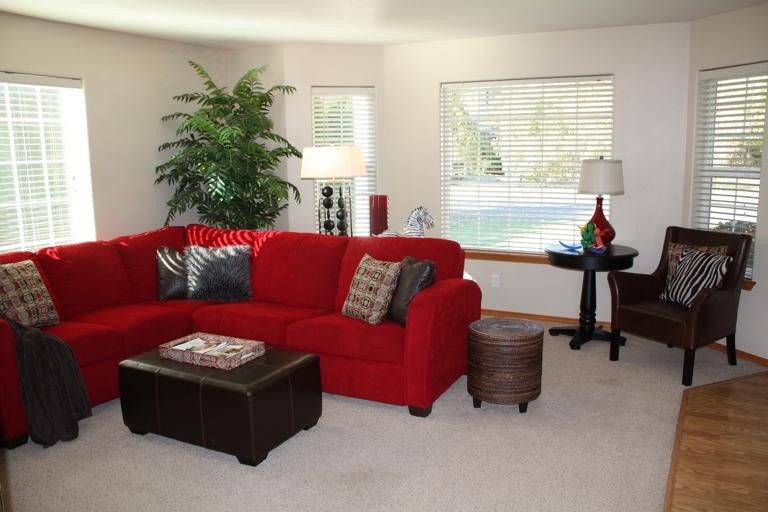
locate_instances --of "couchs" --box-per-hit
[0,225,481,449]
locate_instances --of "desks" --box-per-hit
[545,243,639,349]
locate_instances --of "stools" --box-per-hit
[468,318,545,412]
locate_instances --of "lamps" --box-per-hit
[301,147,364,237]
[578,156,624,246]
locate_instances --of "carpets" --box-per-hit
[0,321,767,512]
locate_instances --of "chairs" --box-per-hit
[609,225,753,385]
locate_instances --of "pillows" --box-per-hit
[155,245,186,300]
[184,246,251,302]
[665,244,729,284]
[342,255,400,324]
[388,256,434,324]
[659,247,733,307]
[0,260,58,329]
